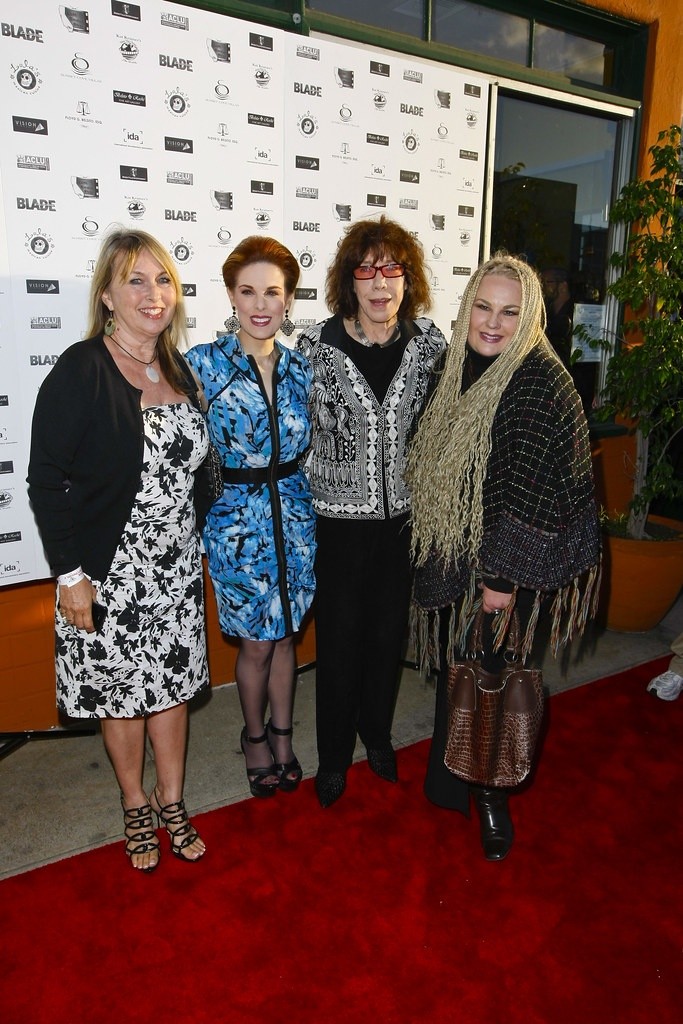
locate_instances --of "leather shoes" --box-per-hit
[477,784,513,862]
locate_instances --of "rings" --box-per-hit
[494,609,500,615]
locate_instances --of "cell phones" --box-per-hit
[57,600,108,630]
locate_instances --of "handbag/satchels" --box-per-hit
[444,606,543,787]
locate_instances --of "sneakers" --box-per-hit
[648,671,683,700]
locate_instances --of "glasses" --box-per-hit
[352,263,406,280]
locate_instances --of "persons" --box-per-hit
[29,229,210,872]
[178,236,315,797]
[403,248,683,857]
[301,214,447,811]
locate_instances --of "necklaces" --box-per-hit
[352,316,399,353]
[106,335,160,383]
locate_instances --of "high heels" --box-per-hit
[151,787,203,861]
[240,725,279,798]
[315,758,354,806]
[266,717,302,792]
[368,743,398,784]
[121,790,160,872]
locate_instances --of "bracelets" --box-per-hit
[58,566,84,590]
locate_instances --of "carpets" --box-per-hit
[0,652,683,1024]
